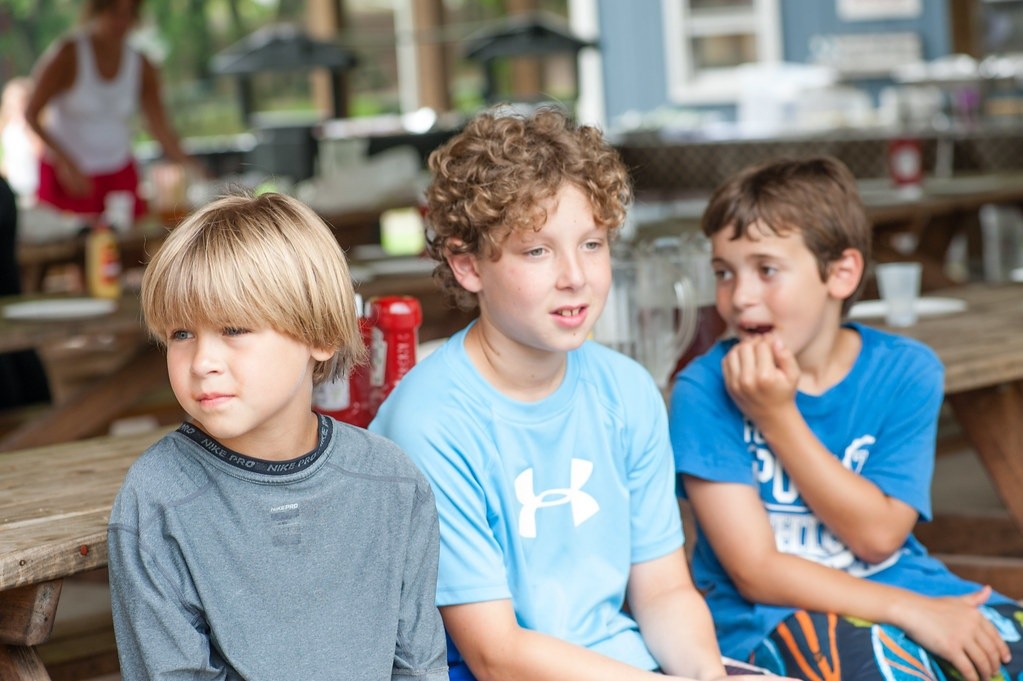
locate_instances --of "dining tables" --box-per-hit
[0,171,1023,681]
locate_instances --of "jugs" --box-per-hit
[593,240,698,387]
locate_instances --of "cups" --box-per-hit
[877,263,922,328]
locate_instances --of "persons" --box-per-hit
[24,0,185,214]
[366,108,797,680]
[108,191,450,680]
[666,154,1022,681]
